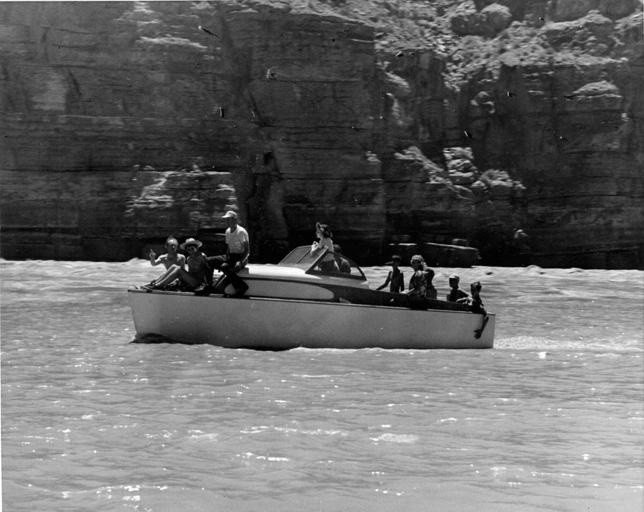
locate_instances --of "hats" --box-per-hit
[181,238,203,250]
[222,211,238,219]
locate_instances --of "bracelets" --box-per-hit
[240,260,243,265]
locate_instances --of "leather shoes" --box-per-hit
[231,284,249,297]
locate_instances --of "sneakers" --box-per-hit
[144,282,165,290]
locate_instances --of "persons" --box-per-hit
[133,237,187,290]
[139,238,209,291]
[466,280,490,340]
[446,274,468,306]
[332,244,352,274]
[310,221,339,273]
[400,254,428,299]
[374,254,405,296]
[193,211,254,298]
[423,268,438,300]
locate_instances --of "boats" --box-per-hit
[124,240,499,352]
